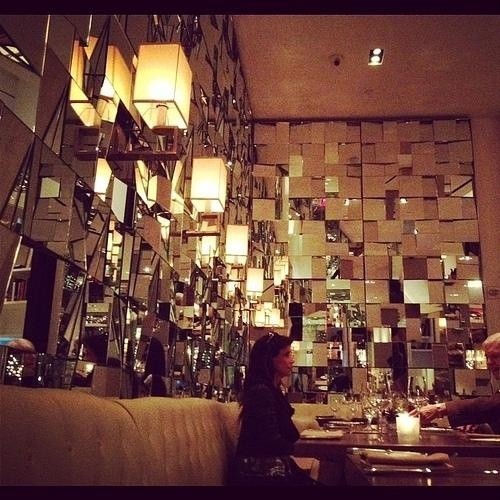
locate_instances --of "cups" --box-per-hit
[396,417,420,445]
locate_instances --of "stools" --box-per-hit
[71,38,125,134]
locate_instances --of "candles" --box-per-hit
[396,412,423,439]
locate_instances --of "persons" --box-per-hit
[4,331,167,397]
[408,333,500,435]
[236,331,343,487]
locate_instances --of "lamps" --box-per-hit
[225,224,248,263]
[254,302,285,327]
[191,156,228,214]
[133,43,194,135]
[245,267,264,300]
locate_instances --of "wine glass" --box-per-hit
[362,398,377,432]
[329,394,344,416]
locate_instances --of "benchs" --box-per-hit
[0,395,499,488]
